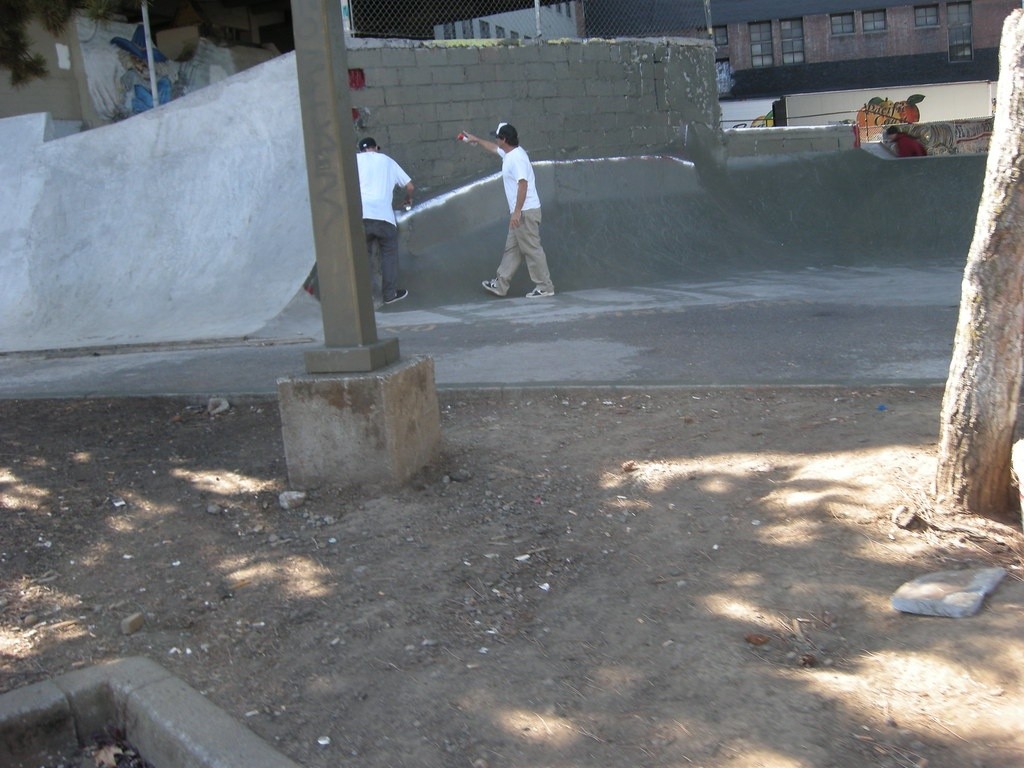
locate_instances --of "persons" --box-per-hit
[992,98,996,115]
[356,136,414,304]
[463,123,555,298]
[887,126,925,156]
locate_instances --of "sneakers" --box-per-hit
[482,278,512,296]
[383,289,408,305]
[525,285,556,300]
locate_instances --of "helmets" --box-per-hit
[489,125,521,145]
[359,138,379,152]
